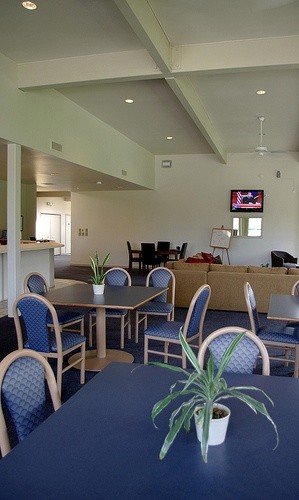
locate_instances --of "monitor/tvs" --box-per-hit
[231,189,264,212]
[271,251,293,267]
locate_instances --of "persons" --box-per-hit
[243,191,259,204]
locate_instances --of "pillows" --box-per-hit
[185,252,222,264]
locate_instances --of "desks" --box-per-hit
[267,294,299,378]
[0,361,299,500]
[39,284,170,371]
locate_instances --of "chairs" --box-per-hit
[126,239,187,268]
[271,251,298,268]
[0,267,299,458]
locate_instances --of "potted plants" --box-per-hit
[87,251,111,295]
[130,325,281,464]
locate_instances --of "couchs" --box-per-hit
[166,259,298,313]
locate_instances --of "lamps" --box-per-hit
[255,117,267,156]
